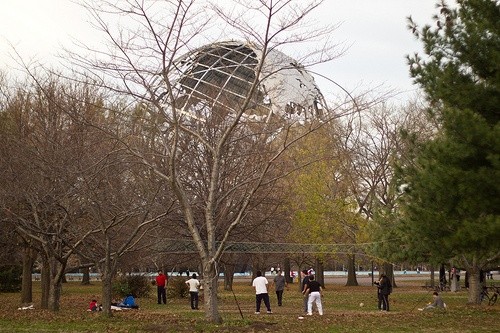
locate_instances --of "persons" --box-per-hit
[251,265,494,318]
[156,269,168,305]
[199,276,205,301]
[185,274,200,310]
[89,294,141,311]
